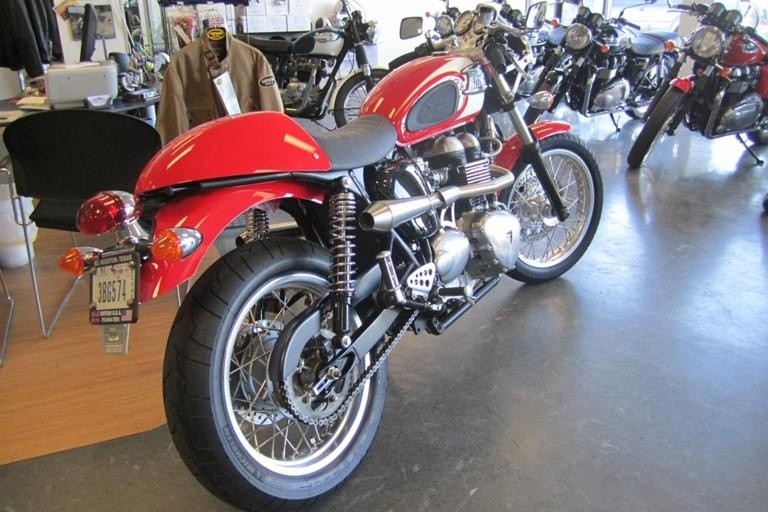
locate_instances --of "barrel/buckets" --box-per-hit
[0,183,39,270]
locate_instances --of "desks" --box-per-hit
[0,90,160,126]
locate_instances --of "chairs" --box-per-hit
[3,106,191,338]
